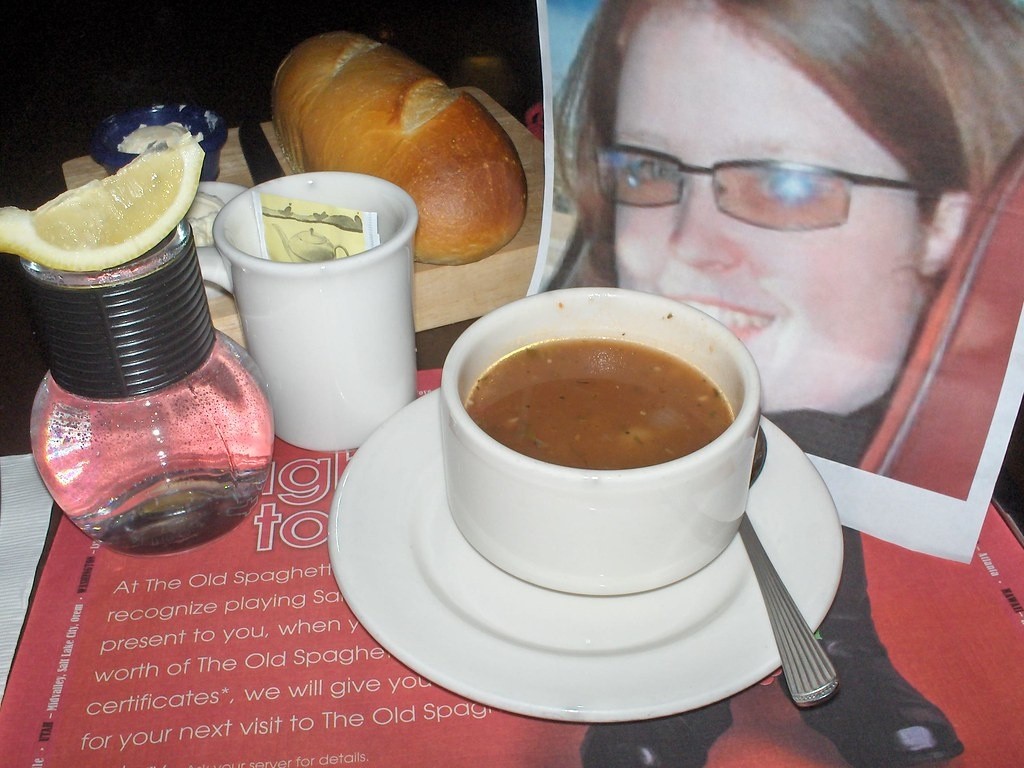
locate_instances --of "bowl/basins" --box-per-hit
[90,102,228,184]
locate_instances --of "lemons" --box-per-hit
[0,133,204,272]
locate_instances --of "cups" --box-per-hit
[215,172,419,451]
[442,287,761,595]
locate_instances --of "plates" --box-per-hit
[329,388,845,723]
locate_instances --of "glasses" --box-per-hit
[593,140,932,237]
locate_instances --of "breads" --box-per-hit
[270,29,527,266]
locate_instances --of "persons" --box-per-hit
[545,0,1023,475]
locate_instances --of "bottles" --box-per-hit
[22,219,274,558]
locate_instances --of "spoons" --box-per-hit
[737,424,838,706]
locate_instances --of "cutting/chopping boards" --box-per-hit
[60,83,551,351]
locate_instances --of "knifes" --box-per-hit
[238,114,284,184]
